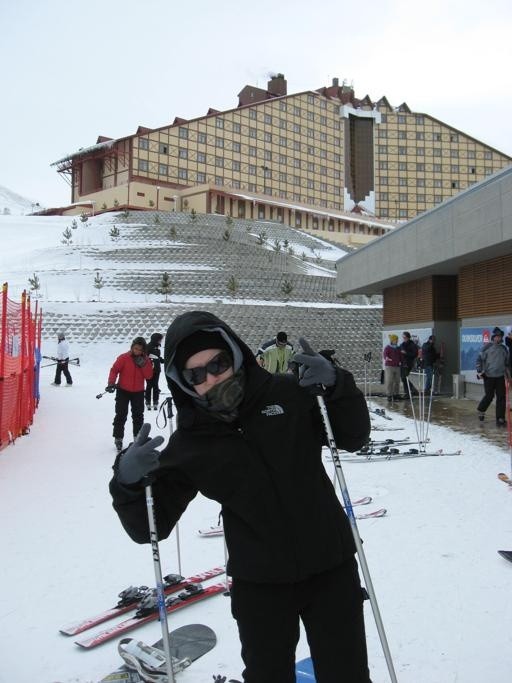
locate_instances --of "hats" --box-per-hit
[58,332,65,336]
[491,327,504,336]
[277,332,287,343]
[173,330,233,377]
[388,334,398,341]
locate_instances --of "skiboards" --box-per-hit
[59,566,233,650]
[43,356,80,366]
[320,407,461,463]
[198,497,387,537]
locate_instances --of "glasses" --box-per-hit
[178,352,234,387]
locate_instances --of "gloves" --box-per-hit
[134,356,145,368]
[116,423,163,484]
[108,384,115,393]
[477,372,483,379]
[294,337,336,388]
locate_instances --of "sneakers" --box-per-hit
[50,382,72,387]
[479,411,507,426]
[146,400,158,405]
[115,437,123,444]
[387,392,418,402]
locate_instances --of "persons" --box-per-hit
[49,333,74,387]
[473,325,512,426]
[144,332,164,410]
[258,330,298,376]
[398,331,419,400]
[420,334,441,396]
[105,336,154,450]
[498,328,512,387]
[107,307,376,682]
[383,332,406,402]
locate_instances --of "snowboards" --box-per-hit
[99,625,217,681]
[229,659,316,682]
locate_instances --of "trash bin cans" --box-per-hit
[452,374,463,399]
